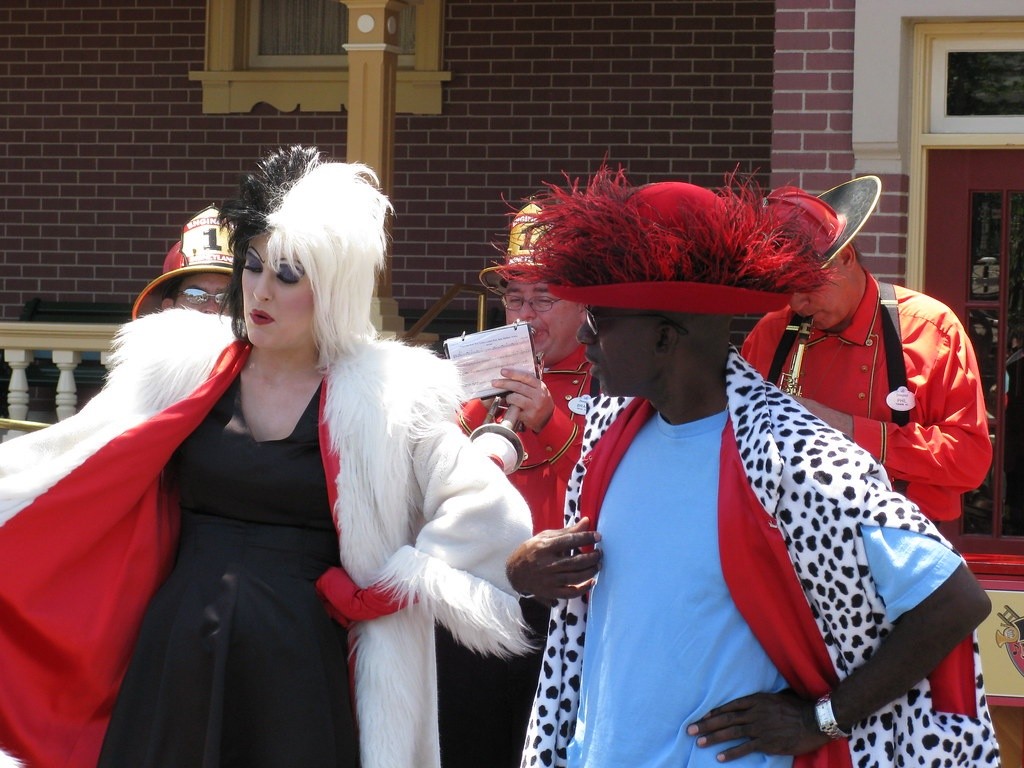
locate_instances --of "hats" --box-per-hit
[494,152,847,314]
[757,175,881,269]
[479,195,557,296]
[132,203,238,321]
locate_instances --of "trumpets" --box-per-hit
[467,350,547,478]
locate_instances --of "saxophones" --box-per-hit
[777,313,814,398]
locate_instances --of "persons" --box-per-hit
[506,180,1000,768]
[131,200,238,322]
[0,165,534,768]
[454,197,637,559]
[739,175,993,531]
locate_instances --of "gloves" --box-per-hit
[317,569,421,630]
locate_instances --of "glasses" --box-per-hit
[501,295,563,313]
[166,287,234,309]
[585,307,689,336]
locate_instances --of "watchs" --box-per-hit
[815,691,853,741]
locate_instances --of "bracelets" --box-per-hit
[504,558,535,599]
